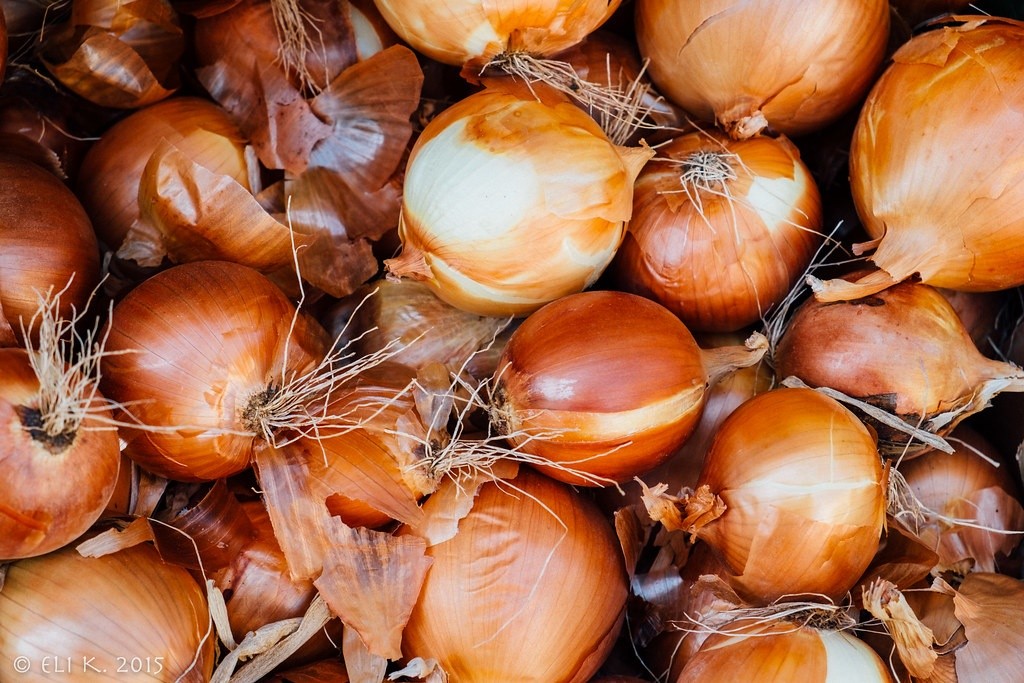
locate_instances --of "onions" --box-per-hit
[0,1,1024,681]
